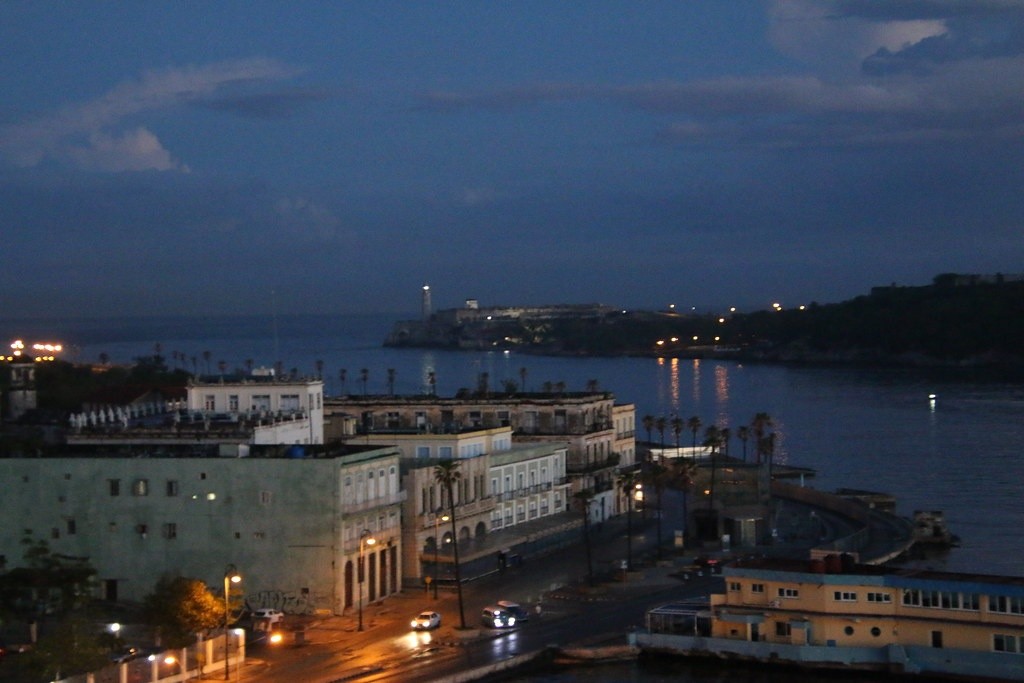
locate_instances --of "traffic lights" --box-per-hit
[419,282,438,319]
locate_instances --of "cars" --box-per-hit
[252,608,285,623]
[411,611,440,631]
[483,601,525,629]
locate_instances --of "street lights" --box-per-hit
[357,531,377,633]
[625,485,648,546]
[161,650,190,681]
[223,563,241,682]
[263,634,284,683]
[102,619,126,653]
[432,505,446,602]
